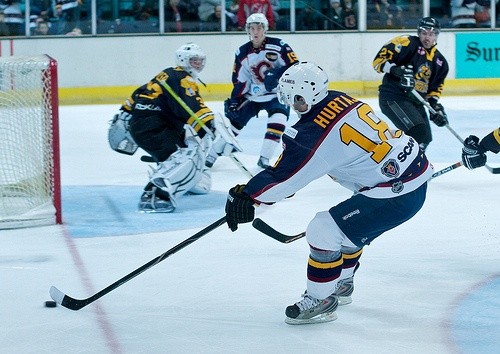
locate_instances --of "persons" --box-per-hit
[225,62,433,319]
[0,0,500,38]
[109,41,244,208]
[206,14,299,169]
[374,18,449,154]
[462,127,500,171]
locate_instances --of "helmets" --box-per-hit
[276,61,328,114]
[245,13,269,36]
[418,17,441,34]
[177,42,207,79]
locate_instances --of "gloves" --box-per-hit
[263,69,279,92]
[429,104,449,127]
[224,98,238,119]
[462,135,486,169]
[390,64,415,87]
[226,184,255,232]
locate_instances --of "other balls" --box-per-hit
[45,301,57,308]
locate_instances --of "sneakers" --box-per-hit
[257,157,272,170]
[305,277,354,306]
[203,155,216,171]
[284,291,338,325]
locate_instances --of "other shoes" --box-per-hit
[138,193,175,213]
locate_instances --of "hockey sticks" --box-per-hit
[50,214,227,312]
[161,78,295,199]
[251,159,463,244]
[412,89,500,174]
